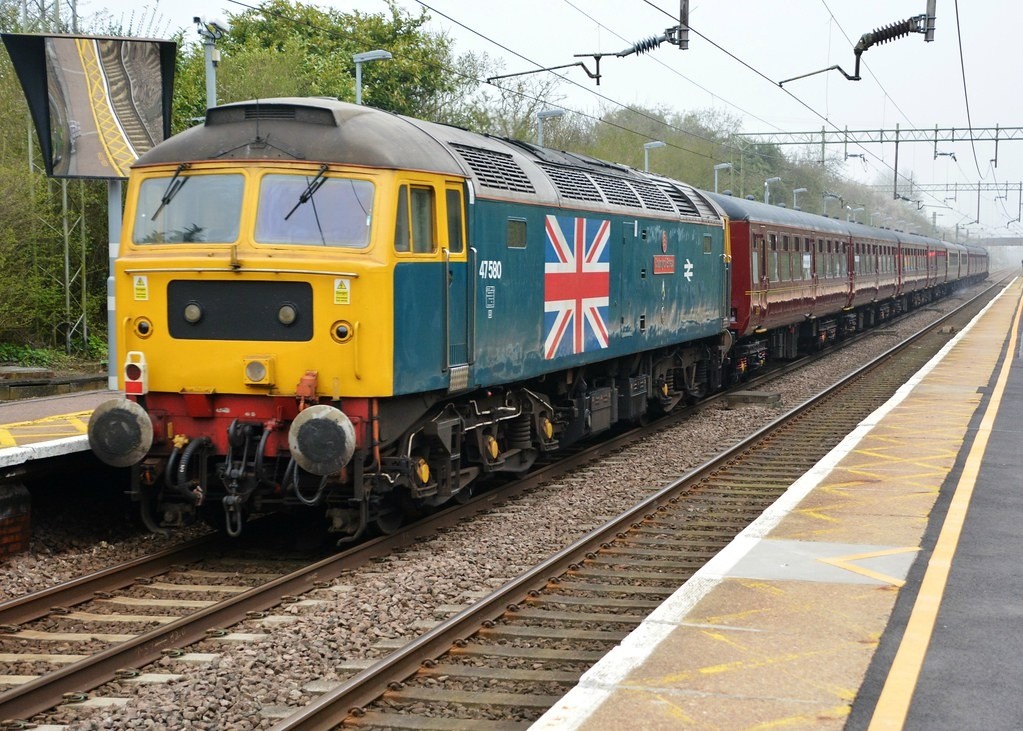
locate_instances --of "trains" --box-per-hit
[87,96,990,547]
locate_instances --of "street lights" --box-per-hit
[353,49,393,104]
[714,162,731,193]
[643,141,667,172]
[537,109,565,146]
[793,187,808,209]
[765,177,780,204]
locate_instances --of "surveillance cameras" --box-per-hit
[209,19,229,35]
[193,14,206,23]
